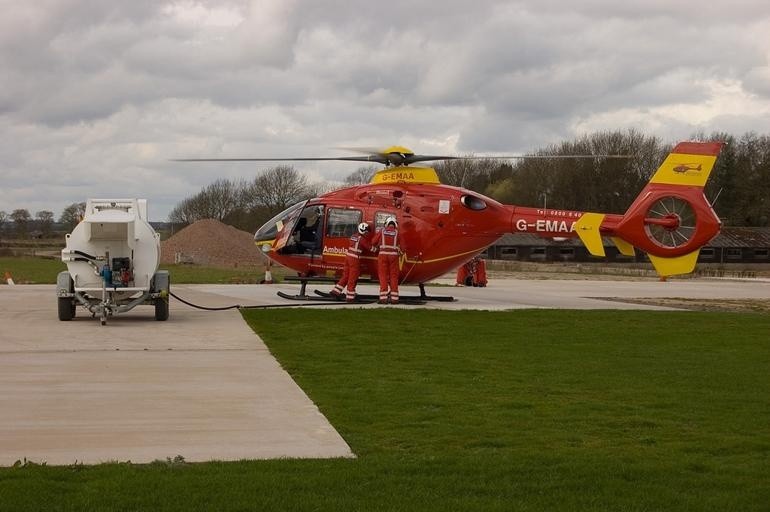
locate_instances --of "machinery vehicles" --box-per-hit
[57,199,171,325]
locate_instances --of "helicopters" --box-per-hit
[166,141,729,305]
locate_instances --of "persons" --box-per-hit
[330,223,378,303]
[371,216,405,304]
[294,207,323,253]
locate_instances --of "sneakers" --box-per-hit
[376,299,401,305]
[330,291,341,301]
[347,297,362,302]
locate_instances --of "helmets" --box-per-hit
[357,222,370,235]
[384,216,398,228]
